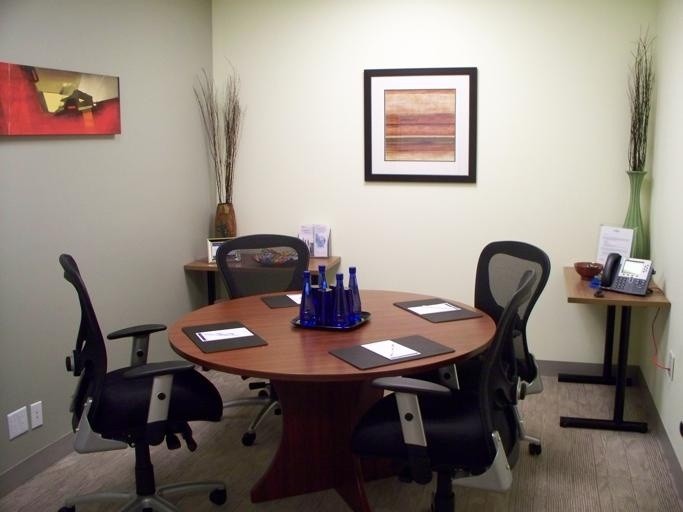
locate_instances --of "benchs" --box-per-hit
[553,264,674,436]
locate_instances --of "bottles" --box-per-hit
[299,264,361,326]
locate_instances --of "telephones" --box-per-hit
[599,253,654,296]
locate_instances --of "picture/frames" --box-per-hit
[362,65,477,185]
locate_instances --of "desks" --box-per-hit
[168,289,496,505]
[183,254,343,309]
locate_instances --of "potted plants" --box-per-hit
[621,20,657,263]
[191,57,251,240]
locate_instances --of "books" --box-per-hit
[295,223,331,259]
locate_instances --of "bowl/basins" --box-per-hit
[573,261,602,281]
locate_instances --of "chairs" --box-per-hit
[215,233,311,298]
[470,234,548,457]
[52,251,230,512]
[358,271,536,510]
